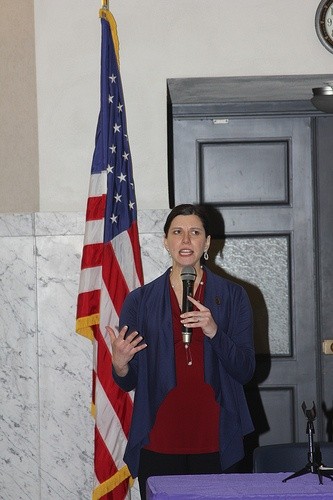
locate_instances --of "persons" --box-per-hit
[105,204,255,500]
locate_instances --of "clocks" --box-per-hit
[315,0,333,53]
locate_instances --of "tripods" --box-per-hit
[282,421,333,485]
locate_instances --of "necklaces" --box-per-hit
[170,265,203,365]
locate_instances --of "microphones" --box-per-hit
[180,265,197,349]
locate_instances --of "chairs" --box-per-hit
[253,441,333,472]
[140,450,221,500]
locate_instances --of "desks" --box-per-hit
[146,471,333,500]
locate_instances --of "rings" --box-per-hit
[197,316,200,322]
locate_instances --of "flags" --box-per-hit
[76,9,144,500]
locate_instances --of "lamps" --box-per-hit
[312,88,333,113]
